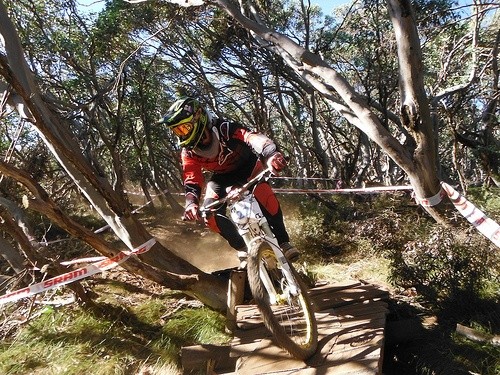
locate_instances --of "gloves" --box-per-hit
[184,203,204,223]
[262,144,286,176]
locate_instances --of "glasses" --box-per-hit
[171,124,191,139]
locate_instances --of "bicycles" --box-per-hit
[181,161,316,359]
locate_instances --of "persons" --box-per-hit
[164,96,299,269]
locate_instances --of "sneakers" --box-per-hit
[237,246,249,269]
[279,242,301,263]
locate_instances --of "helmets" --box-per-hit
[157,97,208,150]
